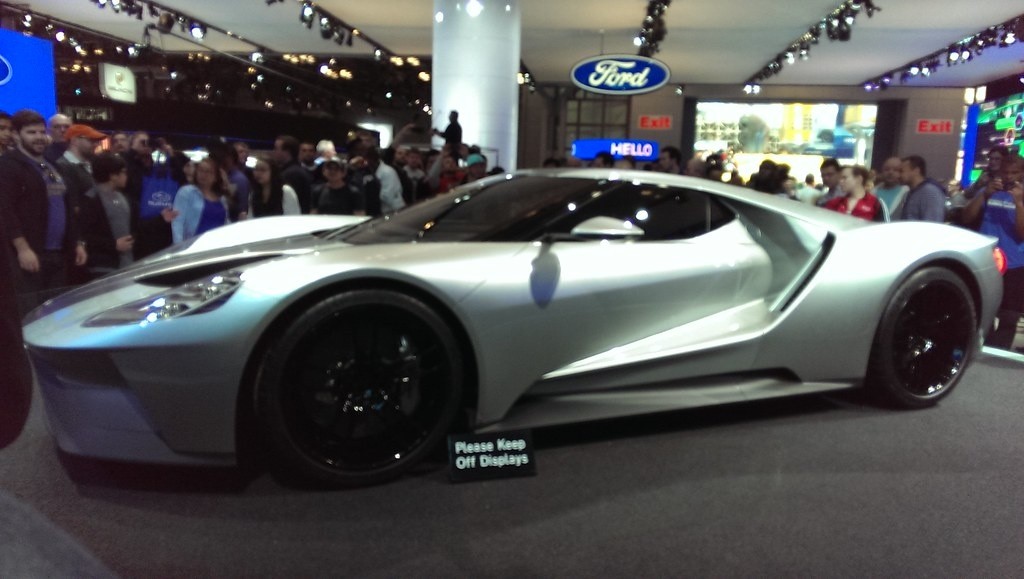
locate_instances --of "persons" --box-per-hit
[0,111,509,313]
[541,146,1024,354]
[0,225,33,446]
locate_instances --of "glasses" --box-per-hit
[139,139,152,147]
[40,161,57,182]
[121,169,129,176]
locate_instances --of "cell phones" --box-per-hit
[998,181,1016,191]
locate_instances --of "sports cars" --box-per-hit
[22,167,1009,489]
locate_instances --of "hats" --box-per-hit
[63,124,107,141]
[313,154,347,165]
[348,156,368,170]
[466,153,486,167]
[316,139,336,153]
[758,159,777,170]
[705,155,722,170]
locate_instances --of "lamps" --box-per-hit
[0,1,1023,92]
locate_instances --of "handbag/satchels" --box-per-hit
[138,150,180,220]
